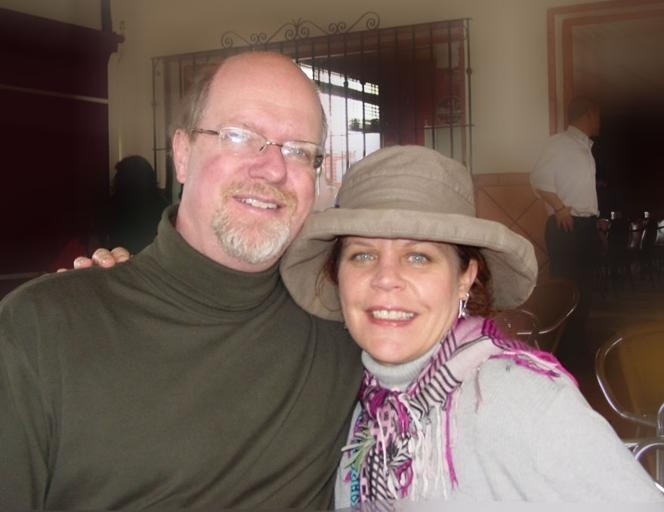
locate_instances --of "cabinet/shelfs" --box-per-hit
[0,8,124,301]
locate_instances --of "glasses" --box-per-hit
[191,125,324,169]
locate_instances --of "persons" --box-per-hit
[107,153,167,256]
[529,95,612,367]
[58,146,664,511]
[0,49,368,511]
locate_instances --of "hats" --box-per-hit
[279,143,539,323]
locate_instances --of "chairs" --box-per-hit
[490,278,581,355]
[596,212,664,297]
[595,322,664,489]
